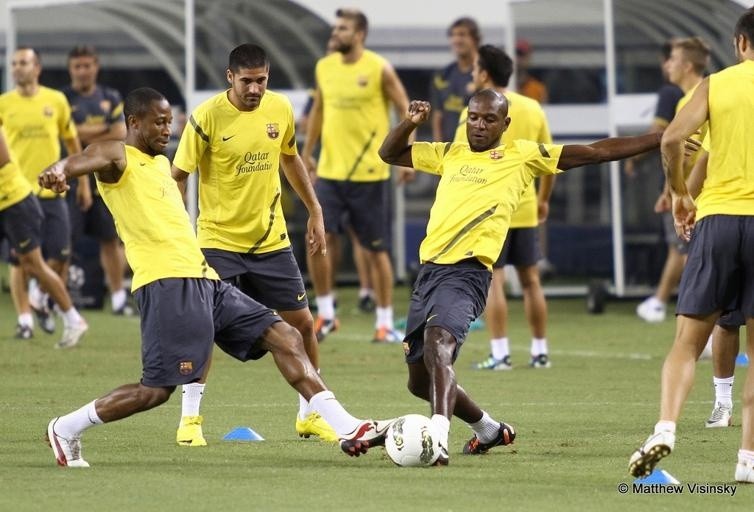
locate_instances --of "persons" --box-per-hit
[0,8,754,484]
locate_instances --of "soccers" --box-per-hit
[385,414,441,467]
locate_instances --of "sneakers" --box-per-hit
[295,408,516,466]
[470,353,512,372]
[112,289,130,316]
[46,416,91,468]
[12,277,89,351]
[636,296,666,322]
[627,431,675,479]
[176,415,208,447]
[529,352,551,370]
[735,462,754,484]
[308,295,406,346]
[705,401,733,429]
